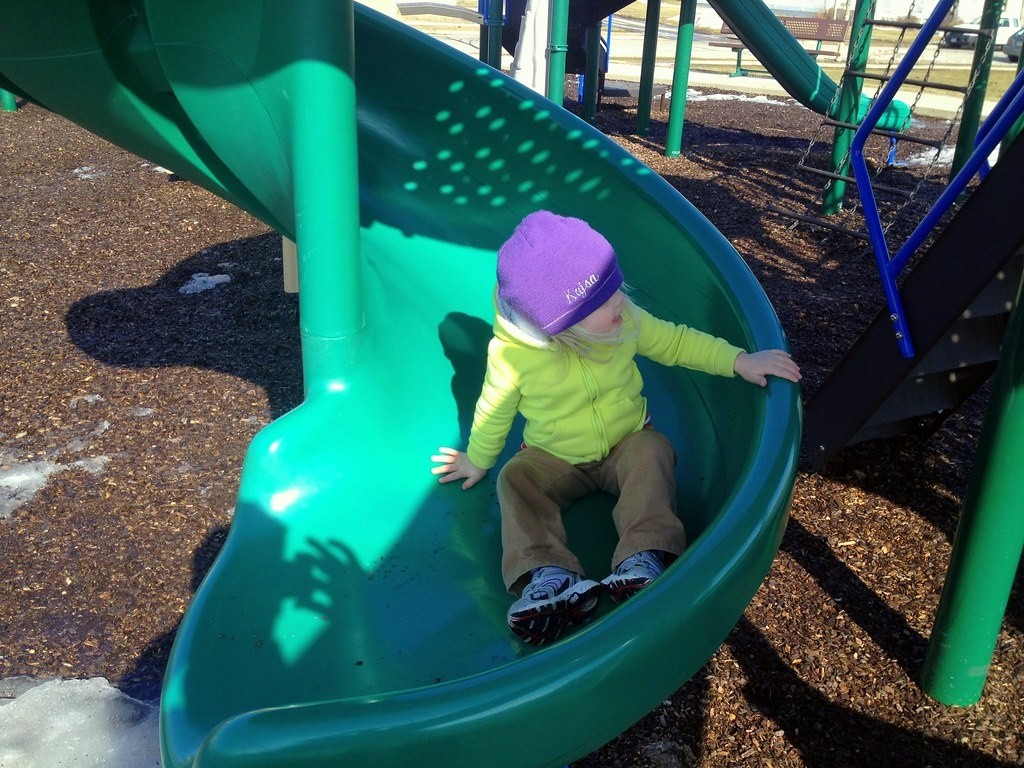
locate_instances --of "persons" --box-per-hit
[430,211,802,646]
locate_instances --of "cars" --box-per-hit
[1002,28,1024,61]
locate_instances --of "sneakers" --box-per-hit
[599,549,664,605]
[507,566,610,647]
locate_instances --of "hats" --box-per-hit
[497,210,622,336]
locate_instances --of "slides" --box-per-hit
[1,0,808,768]
[707,0,914,133]
[501,0,633,75]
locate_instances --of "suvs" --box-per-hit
[945,11,1020,49]
